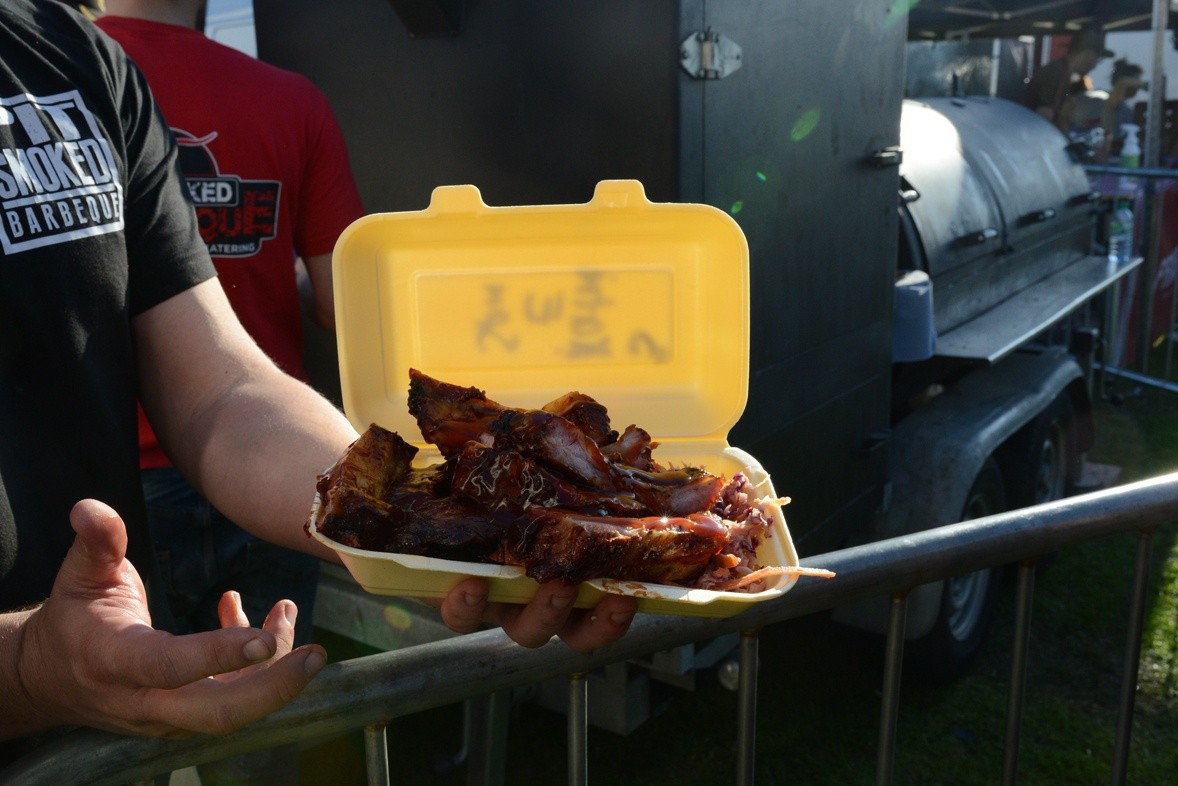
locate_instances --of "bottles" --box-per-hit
[1108,201,1133,265]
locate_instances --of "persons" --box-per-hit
[0,0,636,786]
[1029,24,1113,125]
[91,0,369,786]
[1098,59,1143,125]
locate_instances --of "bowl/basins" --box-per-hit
[307,178,801,617]
